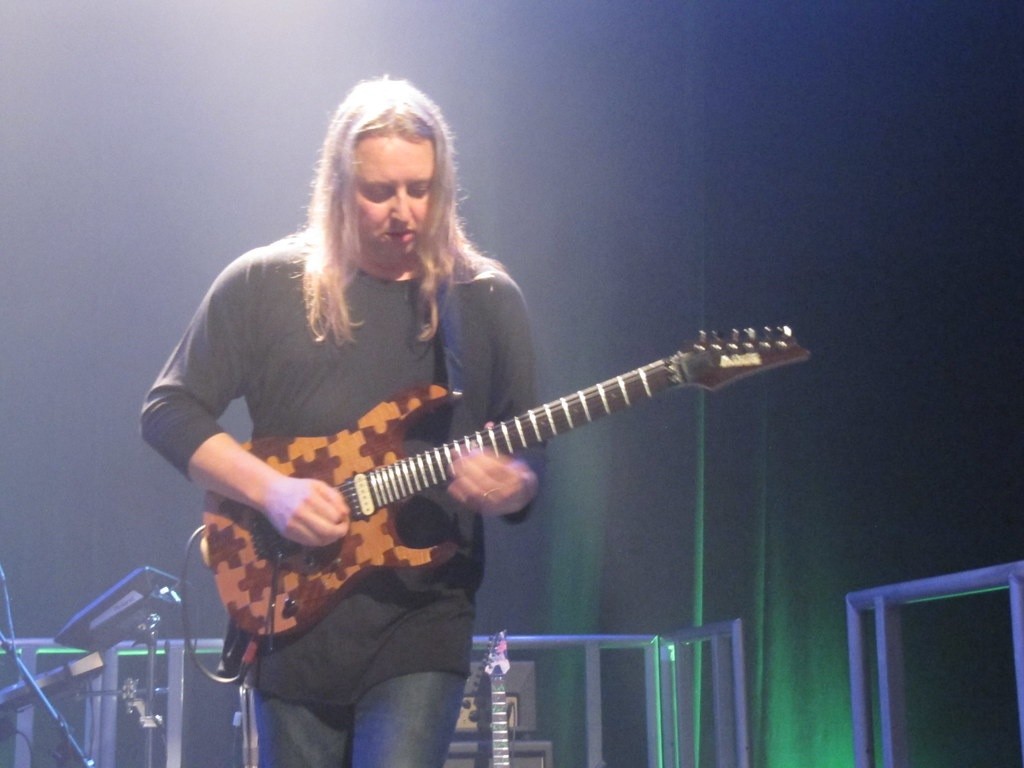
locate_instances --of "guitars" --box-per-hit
[203,321,814,642]
[478,627,515,768]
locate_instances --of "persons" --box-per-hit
[140,76,549,767]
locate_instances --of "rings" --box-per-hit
[482,488,496,498]
